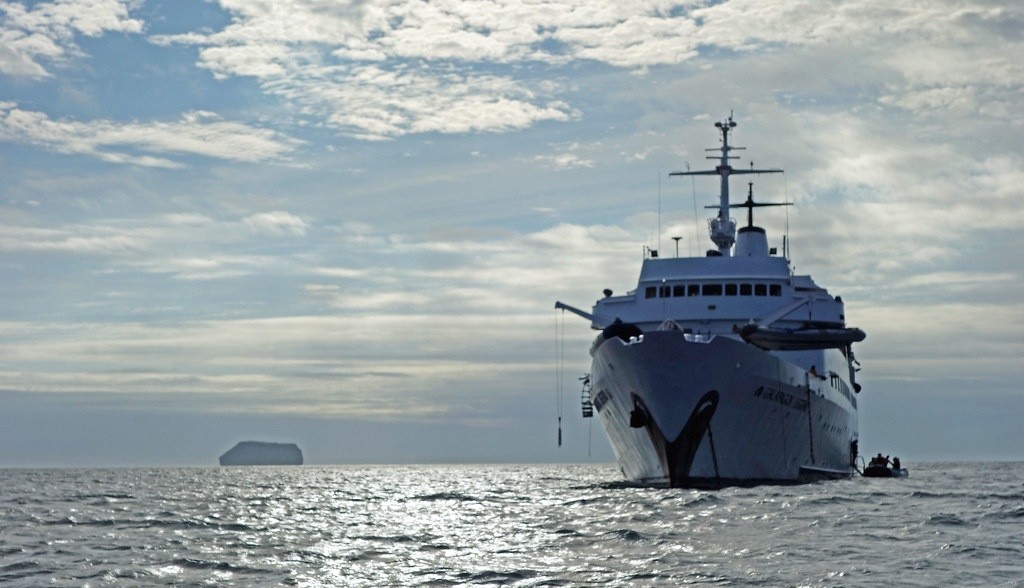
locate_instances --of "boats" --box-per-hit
[553,108,867,489]
[862,463,909,477]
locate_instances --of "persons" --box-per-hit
[851,439,858,463]
